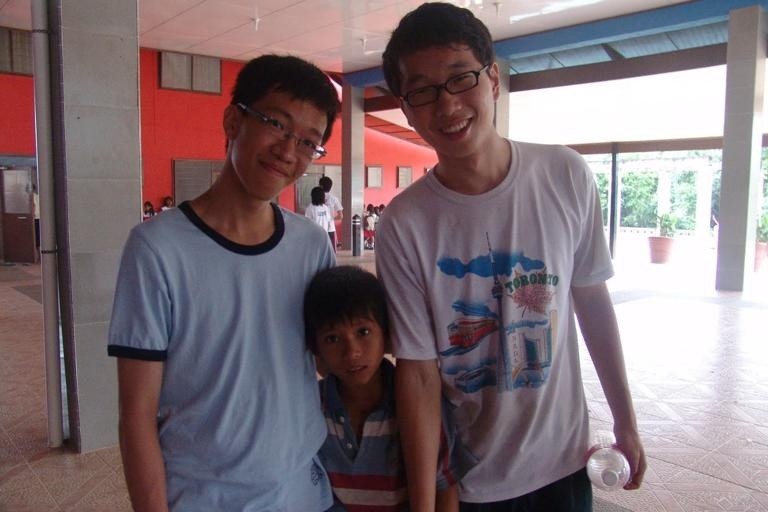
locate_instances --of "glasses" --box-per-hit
[236,102,328,161]
[398,63,489,109]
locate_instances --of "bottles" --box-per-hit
[586,429,631,493]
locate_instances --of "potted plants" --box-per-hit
[753,211,767,272]
[648,213,677,264]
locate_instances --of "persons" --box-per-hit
[304,186,331,241]
[300,264,461,511]
[379,1,646,512]
[363,203,386,250]
[24,183,39,263]
[318,177,345,255]
[159,196,176,213]
[143,199,157,222]
[106,54,340,512]
